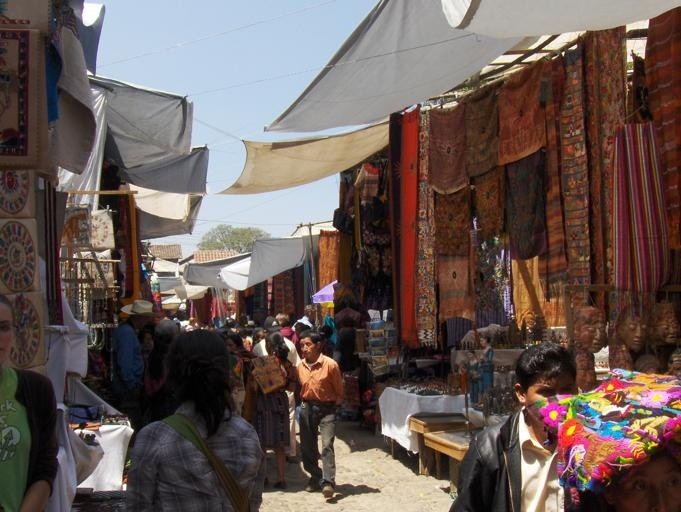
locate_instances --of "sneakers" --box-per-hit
[274,481,285,489]
[322,483,333,497]
[306,469,323,491]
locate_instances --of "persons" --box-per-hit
[109,300,266,512]
[464,349,481,403]
[478,335,496,391]
[579,447,681,511]
[448,341,579,511]
[1,293,60,512]
[235,305,362,498]
[572,301,681,393]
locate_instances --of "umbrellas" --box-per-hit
[311,280,339,303]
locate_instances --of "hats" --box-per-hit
[120,300,159,316]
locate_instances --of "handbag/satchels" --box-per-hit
[252,356,288,396]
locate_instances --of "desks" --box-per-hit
[385,385,468,475]
